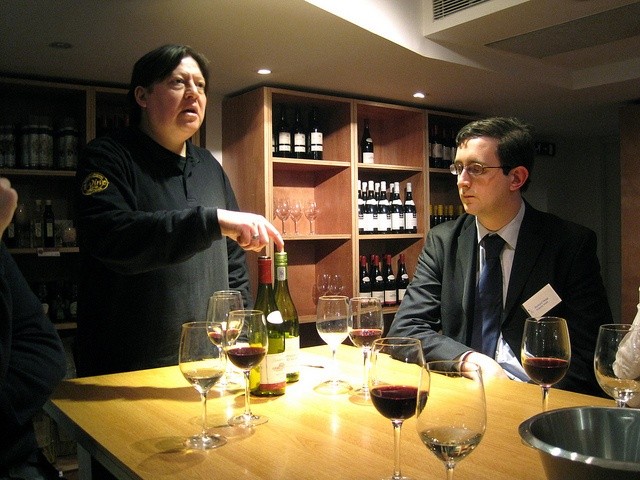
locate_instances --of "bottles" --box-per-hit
[434,205,438,226]
[0,135,5,168]
[360,117,374,163]
[49,127,54,169]
[365,181,377,234]
[29,125,39,170]
[385,255,397,307]
[456,206,464,222]
[389,183,394,233]
[54,294,66,323]
[378,181,389,234]
[404,183,417,233]
[360,256,371,297]
[5,215,19,248]
[59,128,65,169]
[73,130,79,170]
[429,205,433,228]
[392,182,404,234]
[68,284,78,323]
[29,199,45,248]
[361,181,368,200]
[37,285,54,324]
[449,205,454,221]
[45,199,55,247]
[249,256,285,395]
[39,125,49,169]
[307,107,323,160]
[438,204,444,224]
[292,107,308,158]
[276,106,292,158]
[451,128,457,164]
[65,127,74,170]
[358,180,364,234]
[273,253,299,383]
[443,127,451,169]
[444,205,448,221]
[5,134,15,168]
[432,125,443,169]
[397,254,409,305]
[372,256,384,307]
[375,183,381,201]
[22,126,29,169]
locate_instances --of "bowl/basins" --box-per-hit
[518,406,639,478]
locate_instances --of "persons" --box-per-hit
[1,175,70,478]
[385,115,617,398]
[612,280,640,408]
[73,42,284,378]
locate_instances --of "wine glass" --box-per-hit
[276,198,290,236]
[214,290,241,296]
[315,296,353,394]
[416,361,487,478]
[520,317,572,411]
[347,297,386,394]
[291,199,305,235]
[329,274,344,295]
[207,295,245,388]
[317,272,331,296]
[594,324,640,408]
[179,322,229,450]
[304,200,321,235]
[226,310,269,428]
[368,338,429,479]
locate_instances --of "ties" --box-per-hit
[471,236,507,357]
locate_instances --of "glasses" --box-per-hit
[449,163,518,176]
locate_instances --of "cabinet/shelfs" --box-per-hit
[221,86,486,349]
[0,77,200,379]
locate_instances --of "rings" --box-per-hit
[251,233,259,239]
